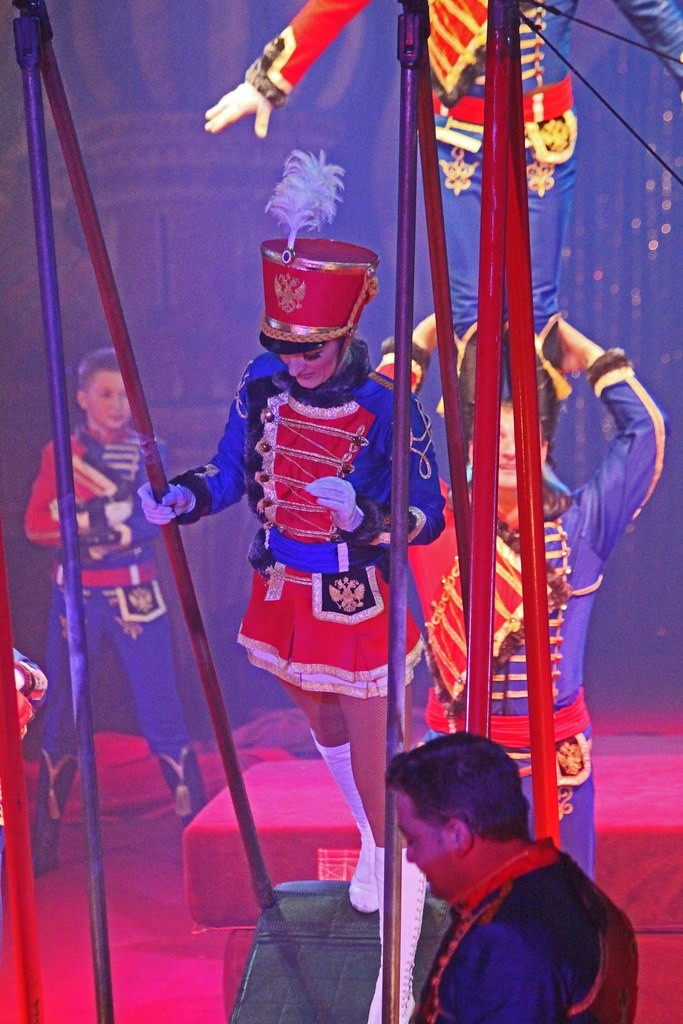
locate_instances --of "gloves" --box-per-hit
[138,481,196,524]
[305,477,363,531]
[49,499,58,520]
[104,501,133,529]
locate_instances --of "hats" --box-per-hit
[259,149,380,353]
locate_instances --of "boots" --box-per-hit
[535,313,572,496]
[448,321,478,498]
[156,746,207,827]
[310,728,378,913]
[367,847,426,1024]
[30,750,76,879]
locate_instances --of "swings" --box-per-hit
[11,0,475,1024]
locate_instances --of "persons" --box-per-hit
[0,648,49,866]
[205,0,683,883]
[382,731,642,1024]
[25,346,206,879]
[136,236,446,1024]
